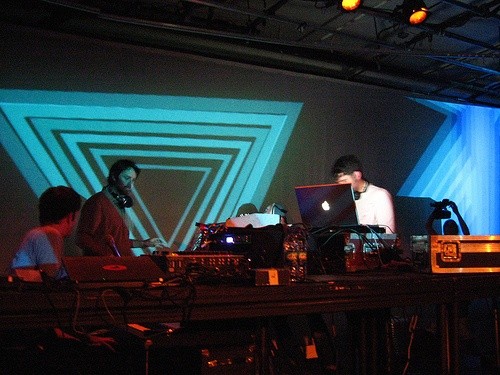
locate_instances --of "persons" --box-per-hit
[12,185,117,351]
[74,159,163,258]
[330,154,395,234]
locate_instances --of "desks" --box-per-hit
[0,290,445,375]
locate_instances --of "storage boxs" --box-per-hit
[411,234,500,274]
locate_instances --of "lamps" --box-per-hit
[340,0,364,13]
[403,0,430,26]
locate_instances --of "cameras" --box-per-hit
[441,199,451,208]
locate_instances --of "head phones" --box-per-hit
[106,182,136,207]
[355,180,369,199]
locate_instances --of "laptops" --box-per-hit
[295,183,379,230]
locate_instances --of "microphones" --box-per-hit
[103,234,121,257]
